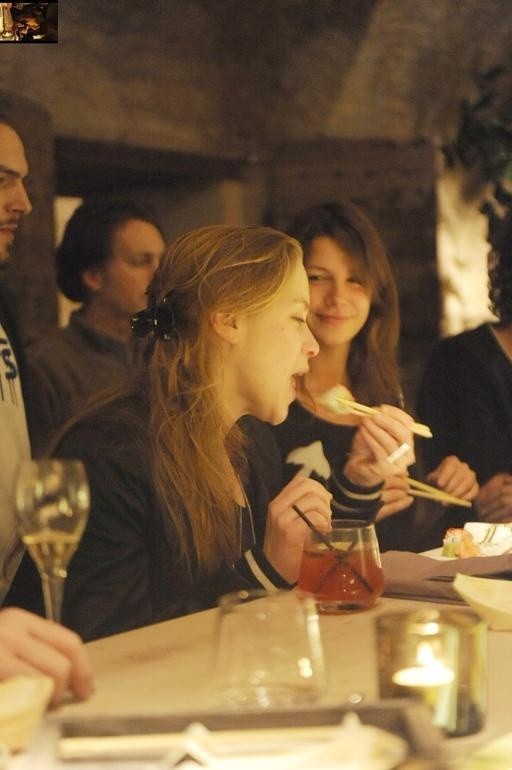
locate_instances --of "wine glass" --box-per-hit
[10,458,92,624]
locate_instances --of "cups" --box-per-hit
[297,519,385,613]
[373,607,487,740]
[207,589,329,713]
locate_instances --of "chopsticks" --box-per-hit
[339,396,433,438]
[404,477,474,508]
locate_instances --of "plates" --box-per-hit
[417,522,512,562]
[450,571,512,632]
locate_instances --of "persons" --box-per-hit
[272,200,479,553]
[421,205,511,521]
[18,196,165,456]
[0,119,95,702]
[3,219,416,643]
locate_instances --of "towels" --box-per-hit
[379,550,512,607]
